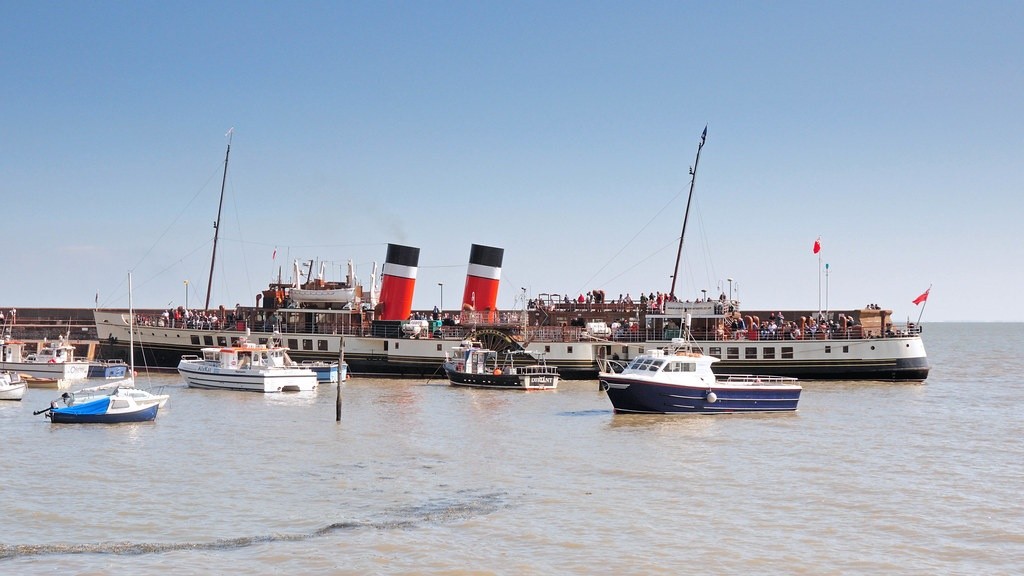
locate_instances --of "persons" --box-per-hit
[134,298,520,331]
[529,289,913,339]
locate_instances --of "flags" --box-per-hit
[813,240,821,253]
[913,290,928,305]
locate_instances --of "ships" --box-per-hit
[91,122,931,380]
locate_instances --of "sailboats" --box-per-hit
[34,273,169,417]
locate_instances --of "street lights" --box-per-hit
[727,278,732,299]
[438,283,443,315]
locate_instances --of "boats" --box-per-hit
[0,309,128,390]
[444,340,561,392]
[297,358,348,384]
[177,326,318,394]
[0,370,25,400]
[591,313,803,413]
[288,287,357,303]
[44,393,160,424]
